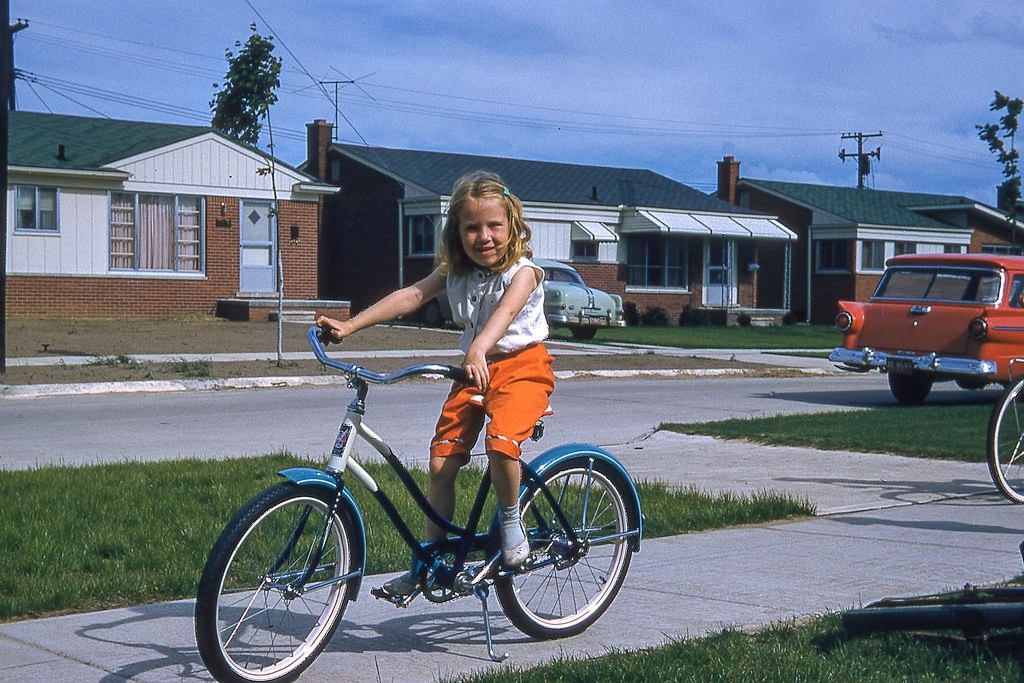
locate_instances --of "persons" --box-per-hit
[317,169,559,595]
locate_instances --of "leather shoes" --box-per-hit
[502,519,530,567]
[383,577,416,597]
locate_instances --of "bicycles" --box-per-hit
[192,326,646,683]
[986,356,1024,505]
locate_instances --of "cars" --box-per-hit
[420,257,617,339]
[829,253,1024,403]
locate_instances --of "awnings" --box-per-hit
[571,206,799,246]
